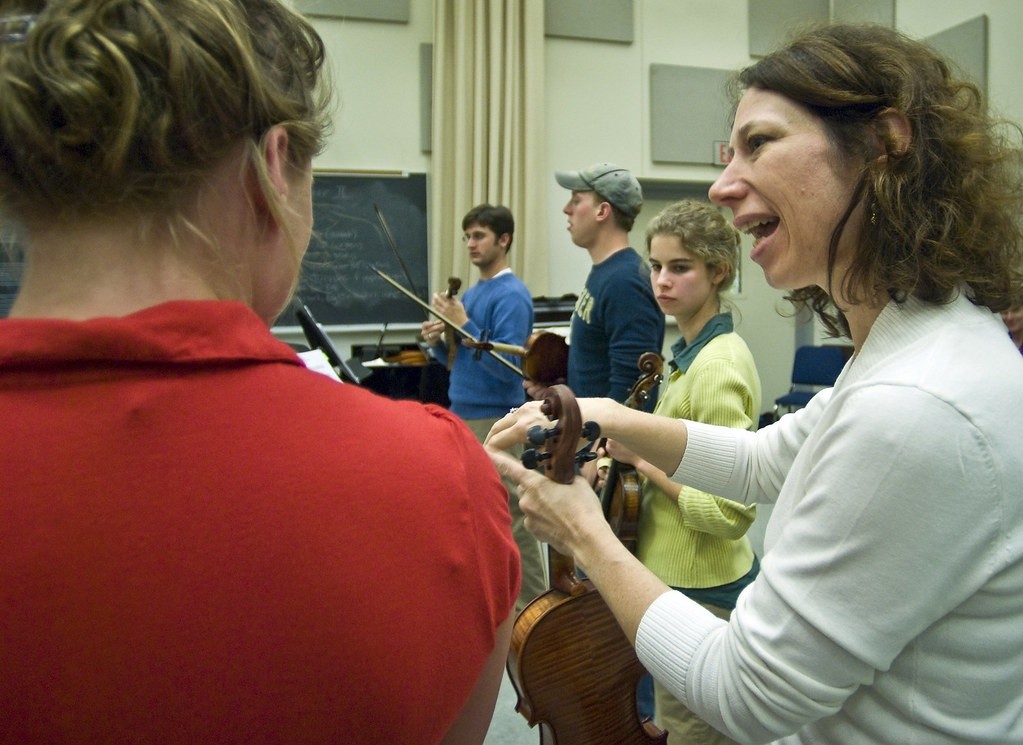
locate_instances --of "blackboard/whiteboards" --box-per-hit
[269,168,433,335]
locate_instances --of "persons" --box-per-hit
[574,199,763,745]
[482,21,1023,745]
[421,202,552,622]
[0,0,523,745]
[553,162,668,722]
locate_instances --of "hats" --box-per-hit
[554,163,643,219]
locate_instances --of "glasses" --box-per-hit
[462,232,504,243]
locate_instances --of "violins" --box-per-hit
[445,275,464,372]
[460,328,569,386]
[504,383,670,745]
[592,350,665,554]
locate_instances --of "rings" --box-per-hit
[509,407,519,414]
[428,333,432,339]
